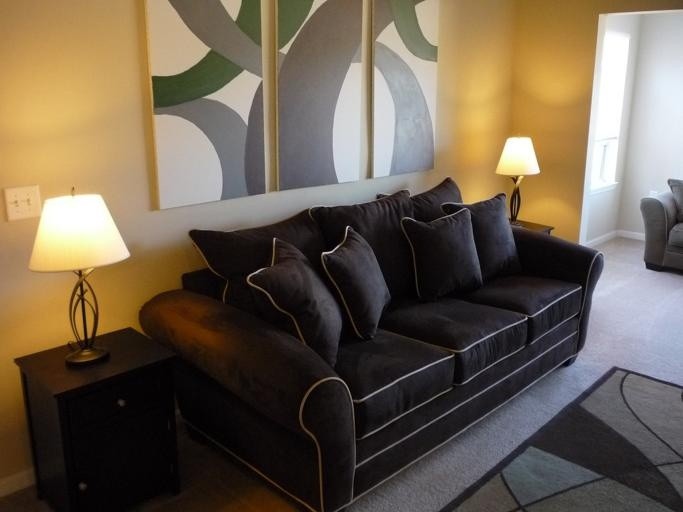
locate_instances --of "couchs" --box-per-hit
[140,226,604,511]
[640,178,682,274]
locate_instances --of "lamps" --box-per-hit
[27,194,131,368]
[496,135,540,226]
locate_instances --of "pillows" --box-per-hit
[440,194,519,287]
[308,190,414,312]
[377,176,464,222]
[248,237,343,369]
[401,208,483,300]
[189,208,325,318]
[321,226,392,340]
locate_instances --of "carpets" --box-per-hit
[440,366,683,511]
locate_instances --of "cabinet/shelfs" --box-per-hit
[13,327,179,511]
[507,218,554,237]
